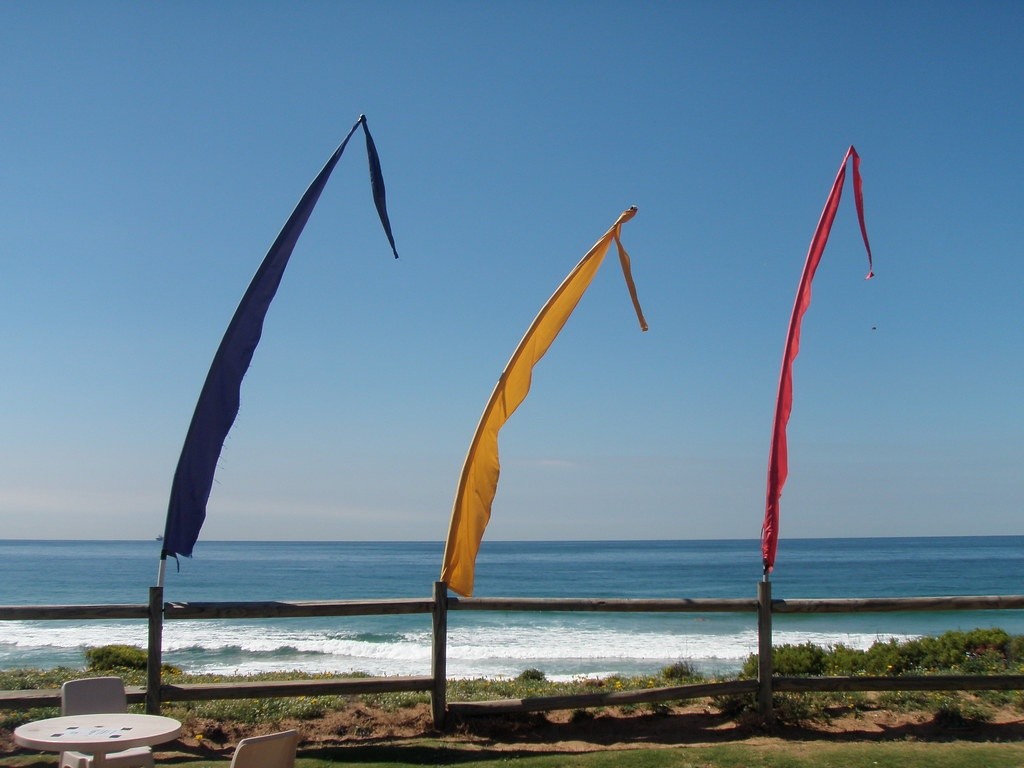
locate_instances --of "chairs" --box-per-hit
[58,676,155,768]
[230,730,298,768]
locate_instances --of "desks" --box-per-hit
[14,715,182,768]
